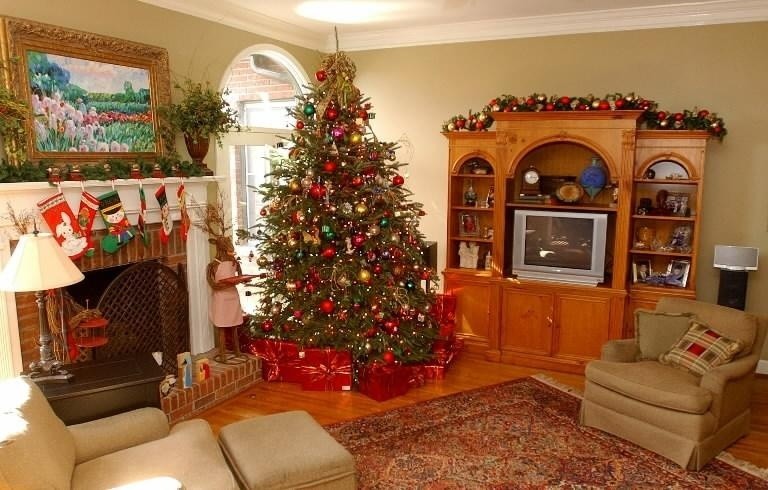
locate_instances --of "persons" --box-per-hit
[671,263,684,281]
[636,263,650,280]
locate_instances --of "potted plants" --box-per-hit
[154,78,244,177]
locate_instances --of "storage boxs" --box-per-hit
[252,295,464,402]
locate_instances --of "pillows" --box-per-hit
[631,306,743,377]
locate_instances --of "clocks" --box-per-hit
[522,167,540,185]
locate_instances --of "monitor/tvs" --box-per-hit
[511,209,608,287]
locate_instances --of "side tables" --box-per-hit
[20,353,168,424]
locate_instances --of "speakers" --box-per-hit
[712,244,759,271]
[717,270,749,311]
[421,241,437,279]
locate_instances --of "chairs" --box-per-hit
[578,294,767,472]
[0,376,243,490]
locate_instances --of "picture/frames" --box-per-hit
[459,213,482,238]
[632,257,689,289]
[0,16,178,171]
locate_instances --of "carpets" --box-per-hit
[322,374,767,490]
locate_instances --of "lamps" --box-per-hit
[1,229,85,385]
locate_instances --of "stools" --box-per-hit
[218,409,354,490]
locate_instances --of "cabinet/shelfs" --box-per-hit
[440,110,710,373]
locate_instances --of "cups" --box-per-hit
[639,197,652,208]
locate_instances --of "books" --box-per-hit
[177,351,191,368]
[195,357,210,382]
[178,361,192,389]
